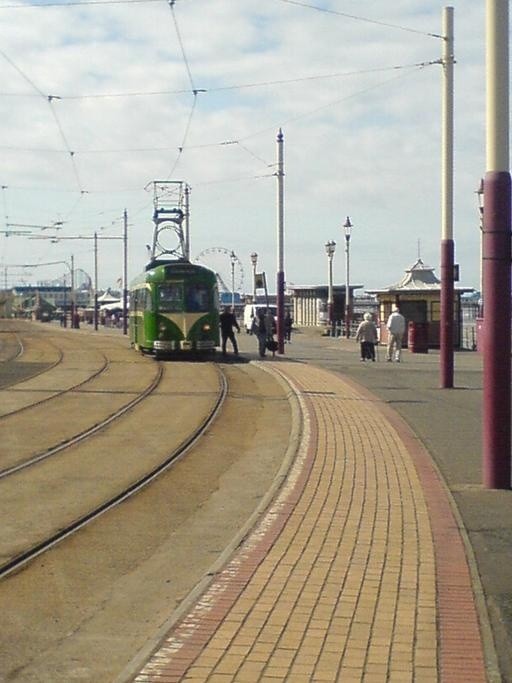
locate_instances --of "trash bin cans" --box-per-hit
[407,321,429,354]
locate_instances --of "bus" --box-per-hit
[128,259,223,359]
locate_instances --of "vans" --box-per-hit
[242,302,278,335]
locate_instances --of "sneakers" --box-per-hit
[385,358,400,364]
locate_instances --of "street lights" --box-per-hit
[324,239,337,337]
[249,250,260,303]
[341,214,354,339]
[229,248,237,312]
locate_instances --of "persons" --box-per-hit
[285,311,293,341]
[356,312,379,361]
[250,307,277,356]
[385,307,405,362]
[220,306,240,354]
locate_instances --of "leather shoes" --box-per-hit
[360,358,376,362]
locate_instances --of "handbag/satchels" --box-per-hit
[264,340,279,352]
